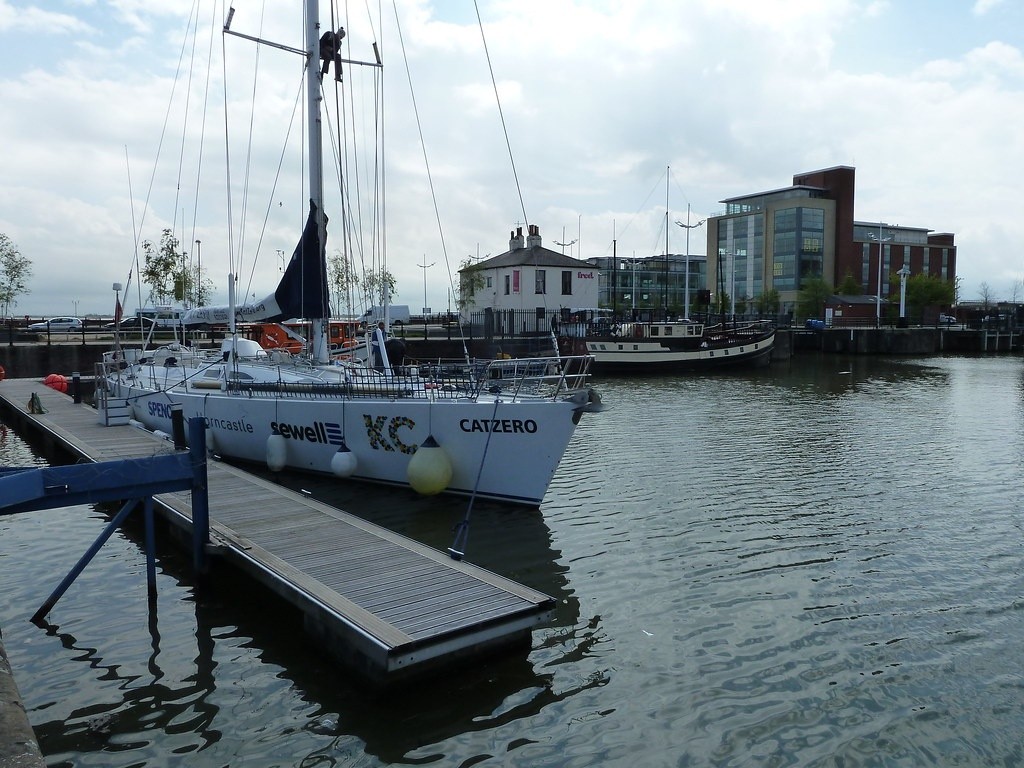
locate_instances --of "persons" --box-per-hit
[371,322,387,373]
[551,314,558,331]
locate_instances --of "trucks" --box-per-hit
[355,304,411,330]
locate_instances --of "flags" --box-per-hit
[114,299,124,323]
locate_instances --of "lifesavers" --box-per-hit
[262,322,289,350]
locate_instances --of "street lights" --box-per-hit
[417,253,435,320]
[183,252,188,306]
[469,244,489,266]
[554,227,579,255]
[868,223,894,328]
[674,203,706,317]
[195,240,202,306]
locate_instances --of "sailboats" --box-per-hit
[556,164,779,370]
[96,0,596,511]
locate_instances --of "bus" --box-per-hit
[135,305,185,331]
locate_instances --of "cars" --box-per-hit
[940,312,957,325]
[106,316,161,334]
[32,317,87,335]
